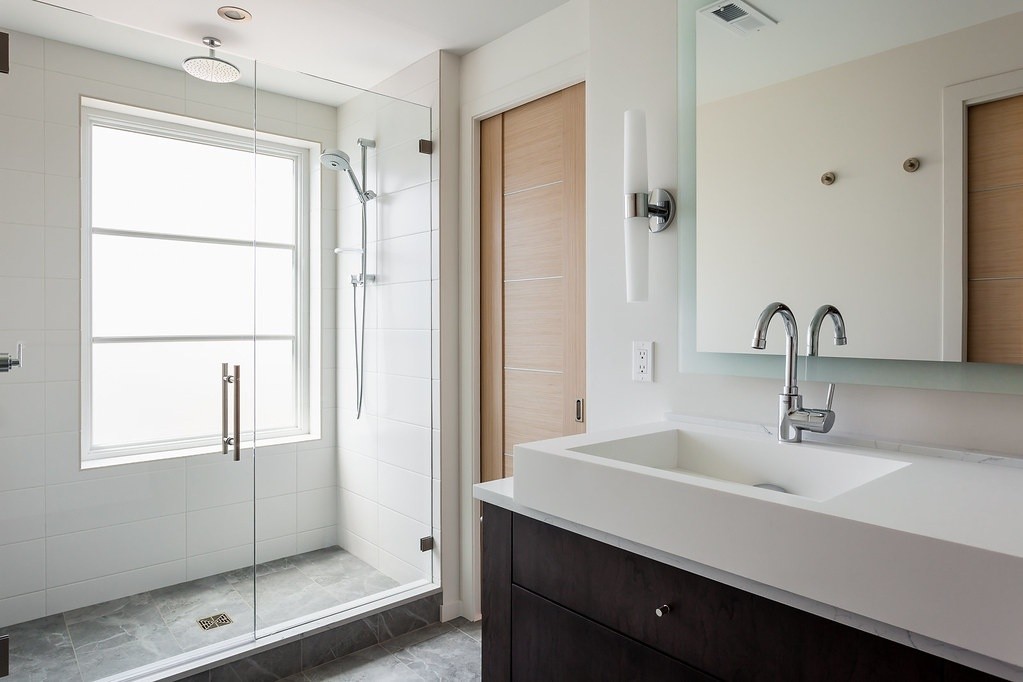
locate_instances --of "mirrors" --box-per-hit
[679,0,1023,395]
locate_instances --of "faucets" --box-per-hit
[807,304,846,356]
[750,302,835,443]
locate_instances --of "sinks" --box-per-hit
[565,426,913,505]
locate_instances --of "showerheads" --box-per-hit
[184,56,242,85]
[321,148,366,203]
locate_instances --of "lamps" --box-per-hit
[621,109,675,234]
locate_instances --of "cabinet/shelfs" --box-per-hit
[477,504,1013,682]
